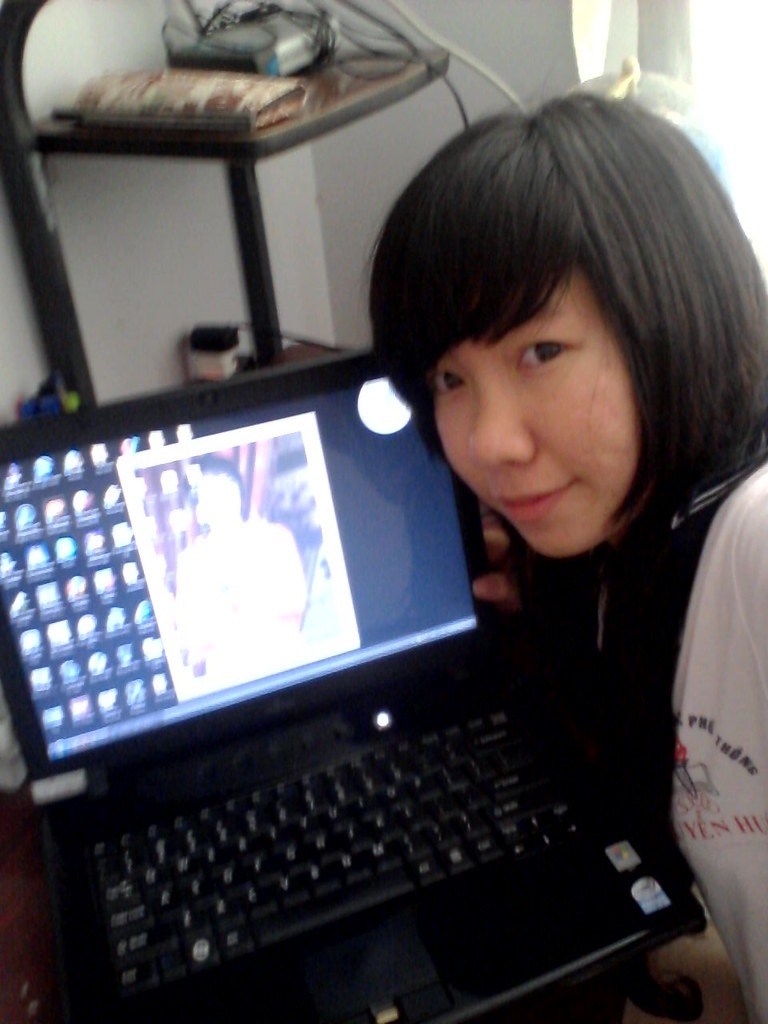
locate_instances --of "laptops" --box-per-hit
[1,345,710,1024]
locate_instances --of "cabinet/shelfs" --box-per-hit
[1,0,451,410]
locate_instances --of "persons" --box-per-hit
[369,93,768,1024]
